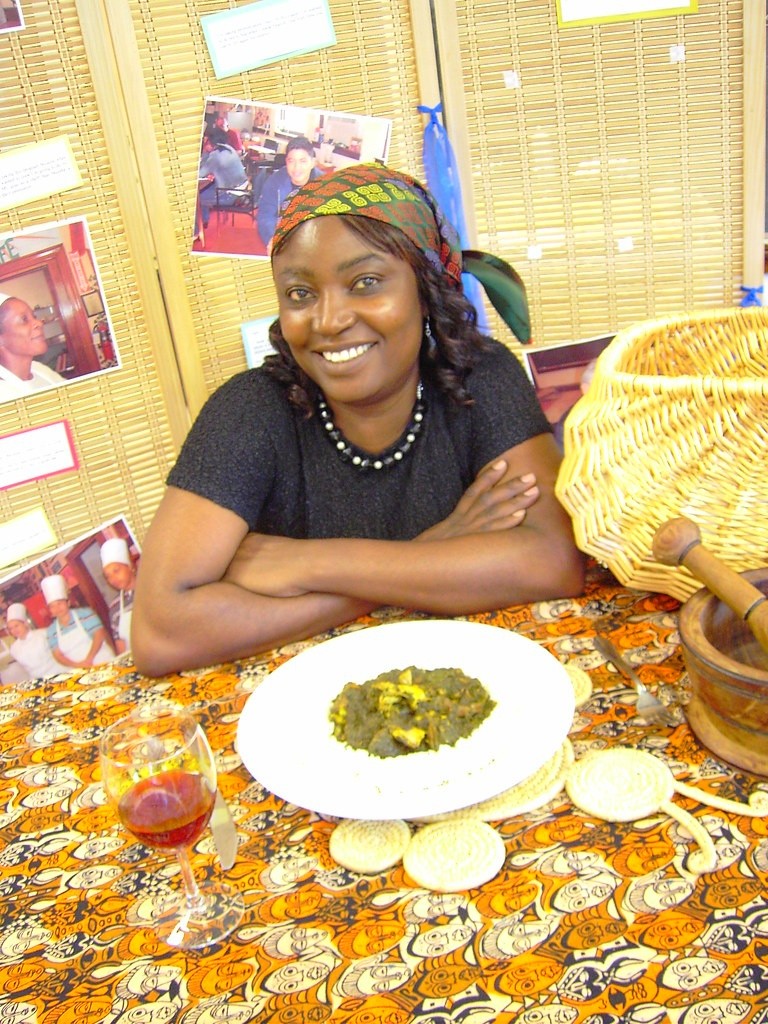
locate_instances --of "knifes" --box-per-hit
[184,723,238,871]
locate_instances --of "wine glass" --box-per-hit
[100,708,244,948]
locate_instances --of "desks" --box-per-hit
[0,565,768,1024]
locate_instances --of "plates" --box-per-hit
[234,620,575,820]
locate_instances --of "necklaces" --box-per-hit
[319,380,438,469]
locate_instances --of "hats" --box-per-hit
[7,603,26,623]
[100,537,131,570]
[41,574,68,605]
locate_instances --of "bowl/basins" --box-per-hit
[678,566,768,738]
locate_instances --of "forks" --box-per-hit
[593,636,679,729]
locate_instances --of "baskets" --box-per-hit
[556,302,768,605]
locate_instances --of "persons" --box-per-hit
[6,538,136,678]
[0,292,67,404]
[194,103,325,246]
[131,165,586,681]
